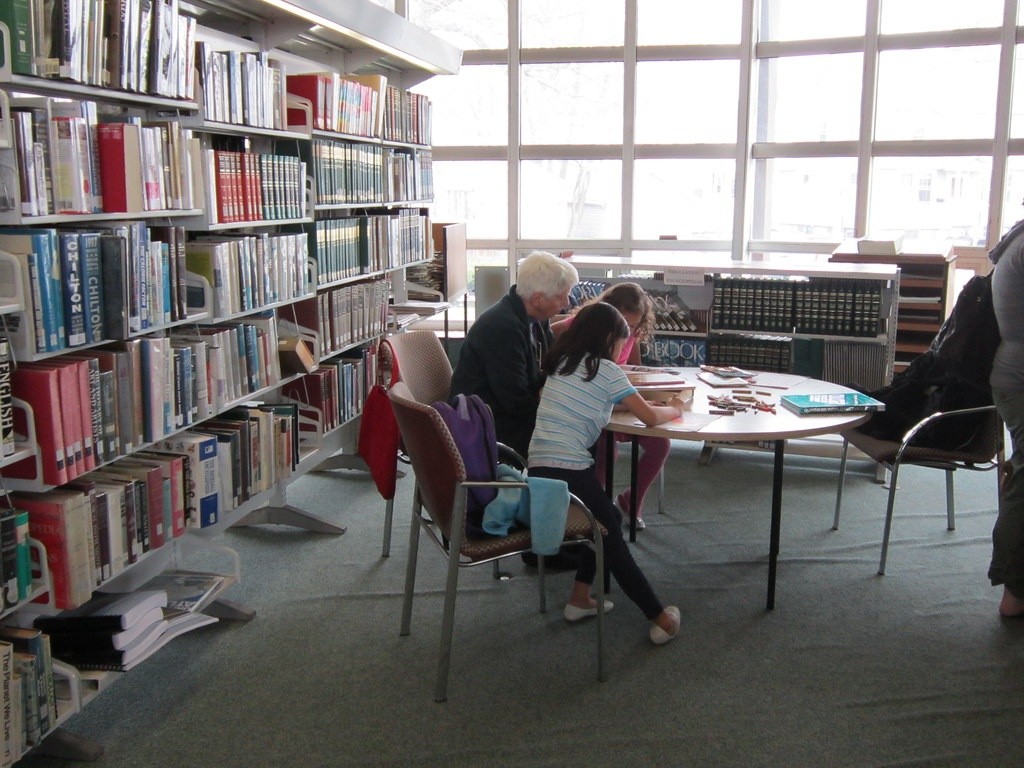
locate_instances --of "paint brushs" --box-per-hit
[706,387,780,416]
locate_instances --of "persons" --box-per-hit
[548,281,670,528]
[526,303,681,645]
[446,250,577,570]
[987,219,1024,619]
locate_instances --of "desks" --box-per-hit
[604,367,871,612]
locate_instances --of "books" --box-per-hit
[558,280,704,332]
[619,339,887,392]
[893,264,945,365]
[706,440,788,450]
[857,231,903,256]
[712,279,884,338]
[0,0,444,768]
[780,390,885,414]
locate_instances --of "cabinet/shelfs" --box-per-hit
[828,238,957,372]
[0,2,464,768]
[518,257,901,480]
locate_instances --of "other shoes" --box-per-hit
[563,600,614,623]
[649,606,680,645]
[614,494,646,530]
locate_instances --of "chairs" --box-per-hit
[381,329,608,702]
[833,371,1007,576]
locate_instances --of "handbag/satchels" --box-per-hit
[357,339,399,500]
